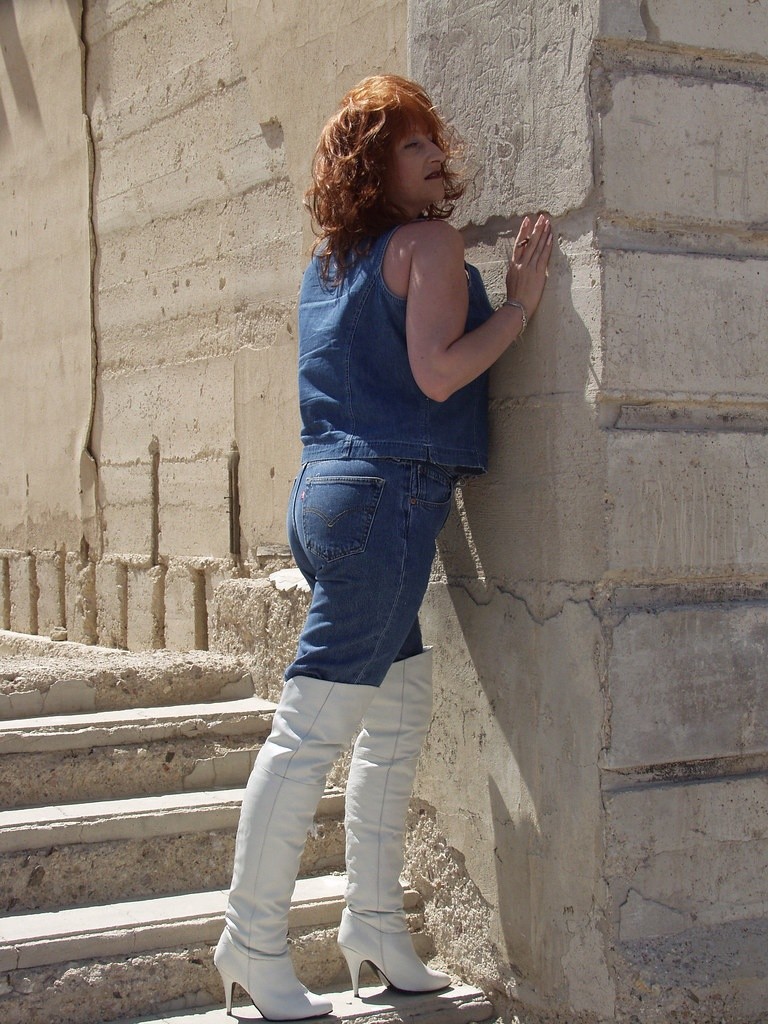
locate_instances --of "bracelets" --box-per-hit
[502,300,528,337]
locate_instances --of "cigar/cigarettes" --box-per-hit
[517,238,529,248]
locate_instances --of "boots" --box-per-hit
[335,646,452,997]
[212,675,386,1020]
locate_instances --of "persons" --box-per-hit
[214,74,554,1022]
